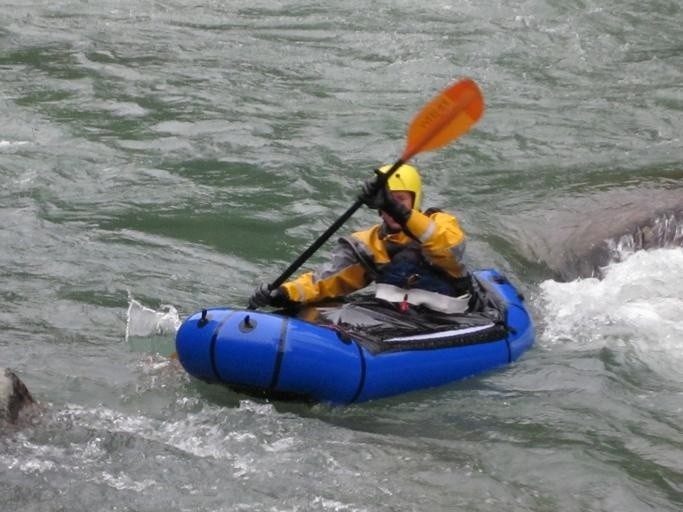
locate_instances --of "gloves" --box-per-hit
[246,279,291,310]
[356,167,414,230]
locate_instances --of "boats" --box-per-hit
[169,262,542,413]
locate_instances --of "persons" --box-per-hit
[246,163,484,325]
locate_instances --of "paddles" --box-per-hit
[245,77,484,309]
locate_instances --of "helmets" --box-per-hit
[372,163,423,217]
